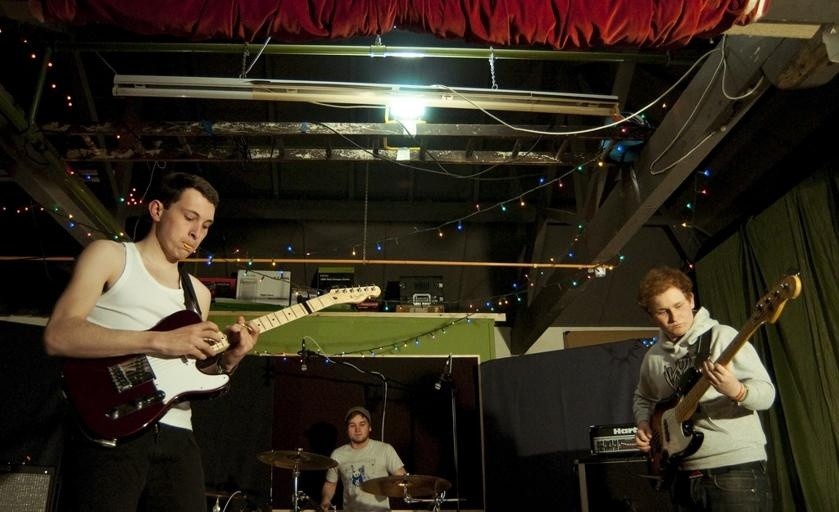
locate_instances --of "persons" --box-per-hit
[318,408,406,512]
[42,173,258,511]
[632,267,777,511]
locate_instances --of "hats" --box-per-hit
[345,406,372,426]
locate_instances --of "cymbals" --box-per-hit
[206,489,246,499]
[258,450,339,470]
[630,465,703,480]
[360,475,452,497]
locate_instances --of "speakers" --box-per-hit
[399,275,444,306]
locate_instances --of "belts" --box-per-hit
[687,461,762,480]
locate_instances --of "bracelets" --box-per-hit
[728,383,747,402]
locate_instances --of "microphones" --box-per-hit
[301,339,307,372]
[434,353,451,390]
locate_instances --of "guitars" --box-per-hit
[647,274,802,472]
[61,285,381,446]
